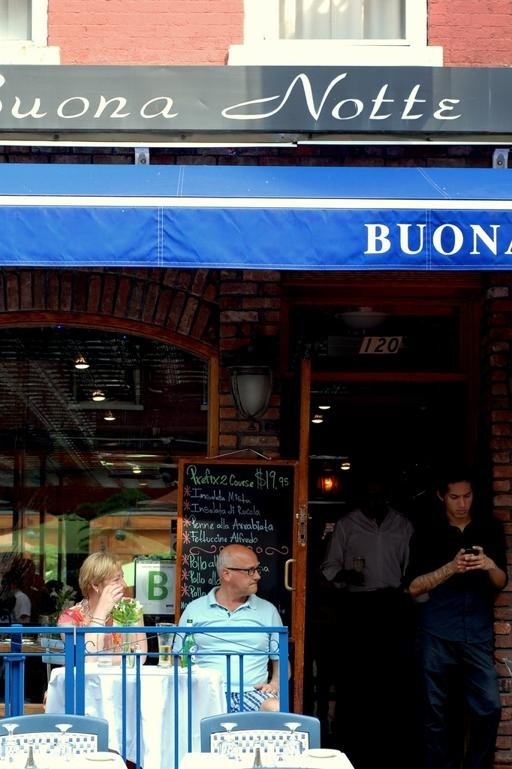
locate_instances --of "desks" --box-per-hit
[0,753,325,768]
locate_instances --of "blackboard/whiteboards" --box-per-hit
[175,459,300,625]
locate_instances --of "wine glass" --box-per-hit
[2,722,20,763]
[54,723,74,763]
[352,554,367,589]
[282,722,304,761]
[218,722,240,763]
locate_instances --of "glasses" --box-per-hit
[228,564,261,575]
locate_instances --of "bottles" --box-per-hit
[24,742,38,769]
[251,742,264,769]
[133,644,143,671]
[182,619,198,668]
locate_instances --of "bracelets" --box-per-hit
[89,620,105,626]
[91,616,107,622]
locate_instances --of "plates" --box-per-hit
[307,748,341,758]
[85,752,115,760]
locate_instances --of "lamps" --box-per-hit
[311,388,352,472]
[74,354,118,423]
[228,332,277,422]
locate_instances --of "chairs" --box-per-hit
[200,712,321,755]
[0,711,108,753]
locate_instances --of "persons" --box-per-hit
[56,552,150,667]
[0,557,65,704]
[403,463,508,768]
[170,544,293,711]
[319,467,419,769]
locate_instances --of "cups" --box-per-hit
[155,622,177,669]
[97,632,113,669]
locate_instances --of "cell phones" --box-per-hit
[463,547,480,557]
